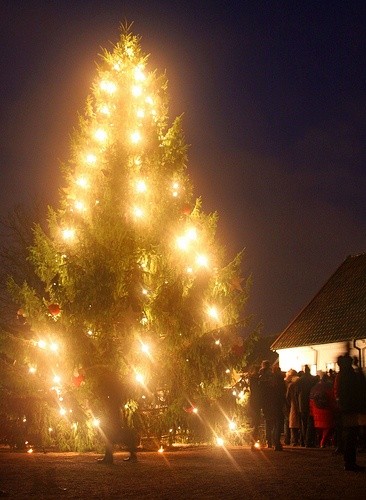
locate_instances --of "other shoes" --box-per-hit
[97,456,113,464]
[0,490,7,497]
[343,464,355,471]
[124,456,136,462]
[285,441,337,449]
[273,444,282,451]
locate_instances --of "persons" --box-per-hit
[244,353,366,472]
[89,376,138,465]
